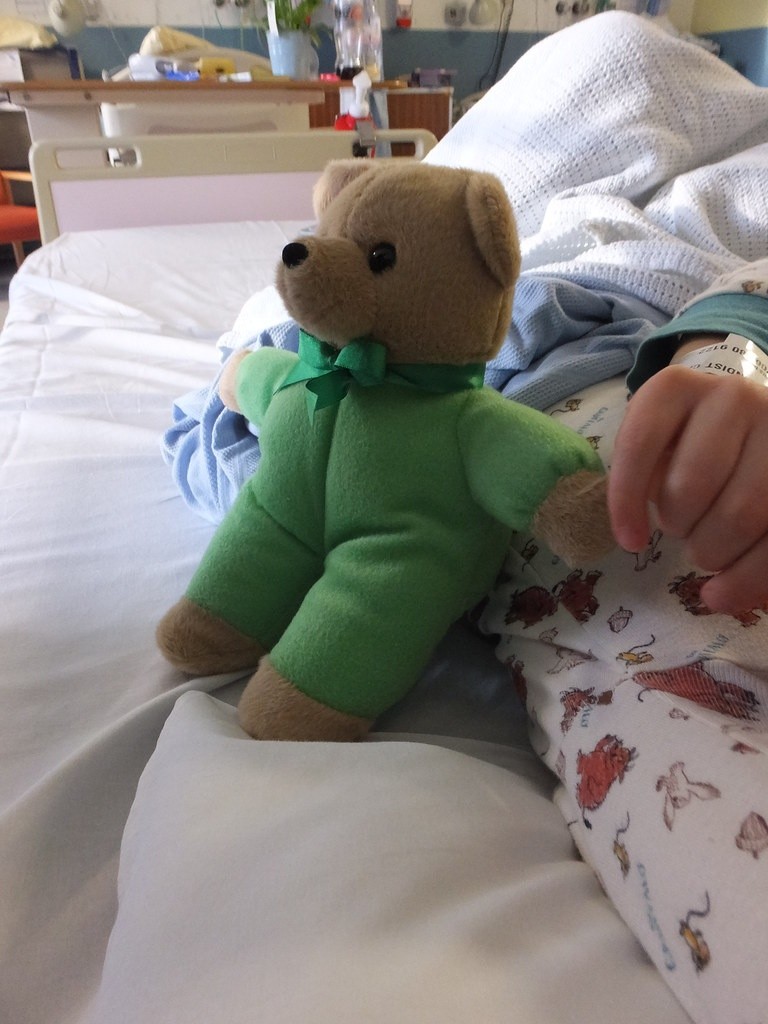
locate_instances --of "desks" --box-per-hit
[1,82,325,168]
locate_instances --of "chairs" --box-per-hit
[0,170,40,269]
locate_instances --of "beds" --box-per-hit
[0,129,690,1024]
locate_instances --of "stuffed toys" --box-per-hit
[156,157,614,742]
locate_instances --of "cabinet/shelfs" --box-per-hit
[309,88,454,157]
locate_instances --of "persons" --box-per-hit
[470,255,766,1024]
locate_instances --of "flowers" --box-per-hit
[214,0,323,46]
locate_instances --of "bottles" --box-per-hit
[337,3,367,79]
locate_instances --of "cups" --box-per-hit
[266,29,320,79]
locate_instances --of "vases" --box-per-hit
[266,30,318,80]
